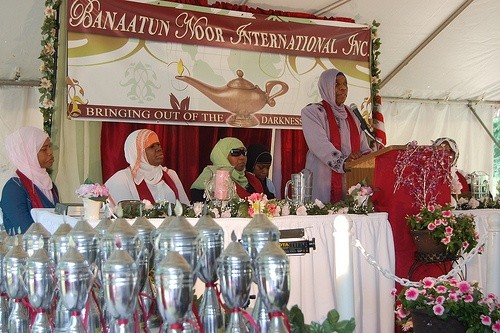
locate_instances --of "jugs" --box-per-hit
[175,70,288,128]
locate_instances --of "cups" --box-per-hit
[284,173,313,209]
[470,171,490,200]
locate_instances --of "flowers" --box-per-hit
[77,179,378,222]
[446,180,500,208]
[391,274,500,333]
[365,20,383,140]
[409,203,488,257]
[37,0,61,133]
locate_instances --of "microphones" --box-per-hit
[350,103,371,132]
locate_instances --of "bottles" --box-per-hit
[488,171,500,201]
[205,165,236,206]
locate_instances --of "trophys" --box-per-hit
[0,213,291,333]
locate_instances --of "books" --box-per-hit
[55,203,104,218]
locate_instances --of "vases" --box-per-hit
[409,309,468,333]
[412,231,444,252]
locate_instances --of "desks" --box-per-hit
[451,208,500,300]
[30,206,396,333]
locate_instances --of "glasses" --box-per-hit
[231,149,248,156]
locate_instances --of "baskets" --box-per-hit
[409,229,459,263]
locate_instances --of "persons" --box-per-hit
[190,137,269,204]
[245,145,276,199]
[104,129,191,207]
[0,128,61,236]
[435,138,469,195]
[301,69,372,205]
